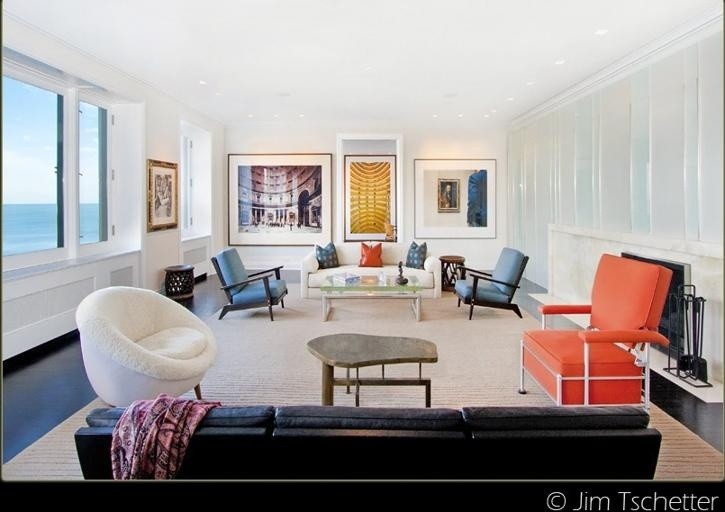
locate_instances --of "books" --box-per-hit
[335,273,379,286]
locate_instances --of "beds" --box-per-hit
[320,275,423,322]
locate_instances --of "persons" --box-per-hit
[442,184,452,208]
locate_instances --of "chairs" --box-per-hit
[520,253,673,417]
[75,286,218,409]
[453,247,529,320]
[210,247,288,322]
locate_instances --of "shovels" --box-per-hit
[692,296,708,382]
[680,294,694,371]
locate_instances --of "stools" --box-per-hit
[164,264,195,300]
[439,256,466,292]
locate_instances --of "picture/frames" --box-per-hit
[413,159,497,239]
[227,153,332,246]
[146,159,178,234]
[343,154,397,242]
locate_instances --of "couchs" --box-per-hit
[74,405,663,480]
[300,241,442,299]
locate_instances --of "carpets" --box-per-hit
[2,283,724,481]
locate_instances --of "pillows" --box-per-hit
[490,247,524,295]
[84,406,650,431]
[358,242,383,267]
[315,241,340,270]
[216,248,248,297]
[406,241,427,270]
